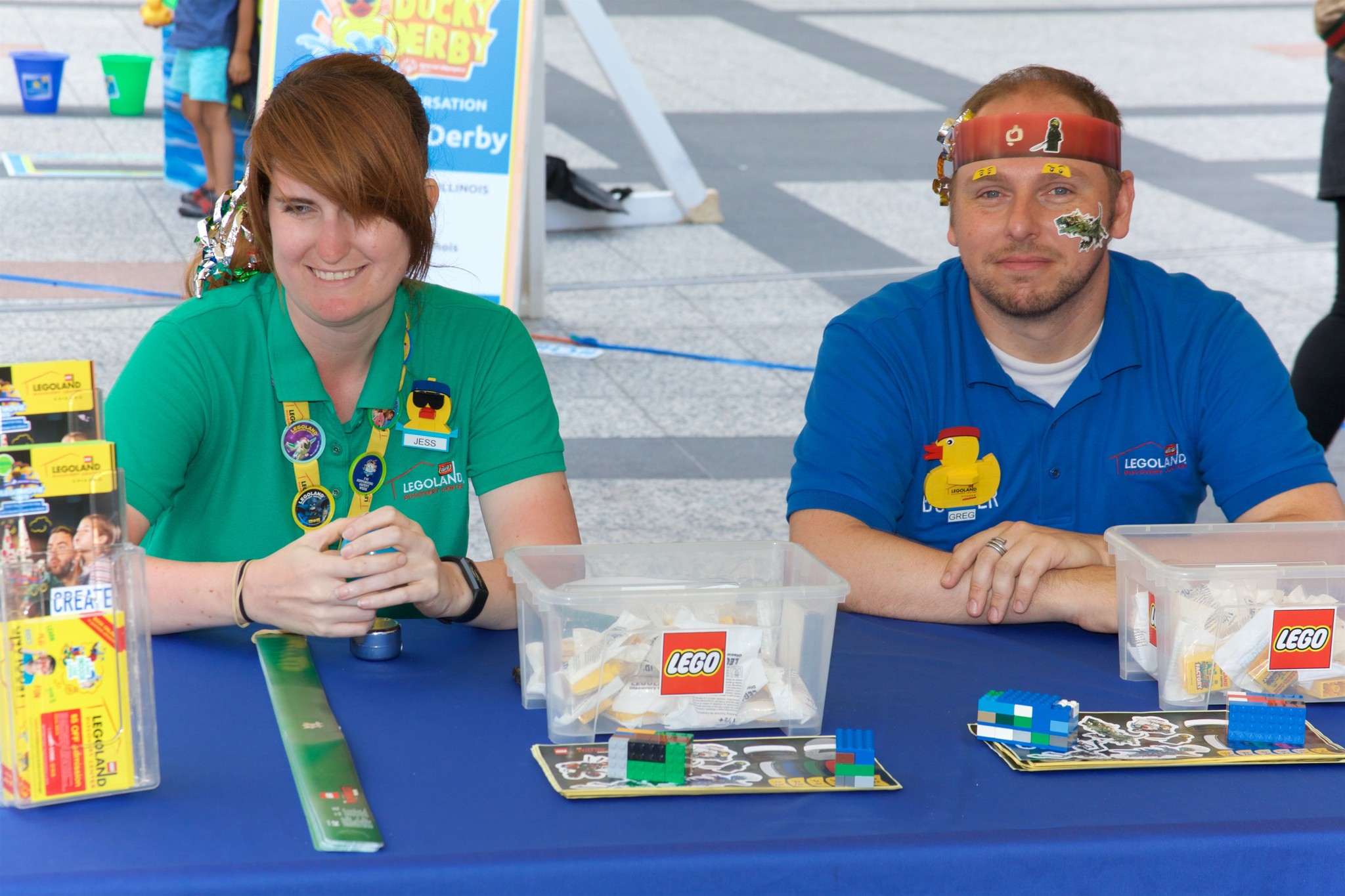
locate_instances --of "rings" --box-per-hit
[984,536,1008,557]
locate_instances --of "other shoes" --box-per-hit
[178,183,221,216]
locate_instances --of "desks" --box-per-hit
[0,610,1345,896]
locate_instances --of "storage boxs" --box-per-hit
[1104,520,1345,710]
[504,541,851,745]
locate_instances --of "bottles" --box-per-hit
[339,529,404,661]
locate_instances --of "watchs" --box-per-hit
[440,551,489,625]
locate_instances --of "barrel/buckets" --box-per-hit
[100,54,151,116]
[11,51,68,114]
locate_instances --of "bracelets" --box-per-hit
[229,559,253,629]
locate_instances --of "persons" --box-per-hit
[58,431,87,444]
[1290,0,1345,460]
[787,66,1345,636]
[166,1,255,217]
[17,514,122,619]
[73,52,583,639]
[23,652,56,684]
